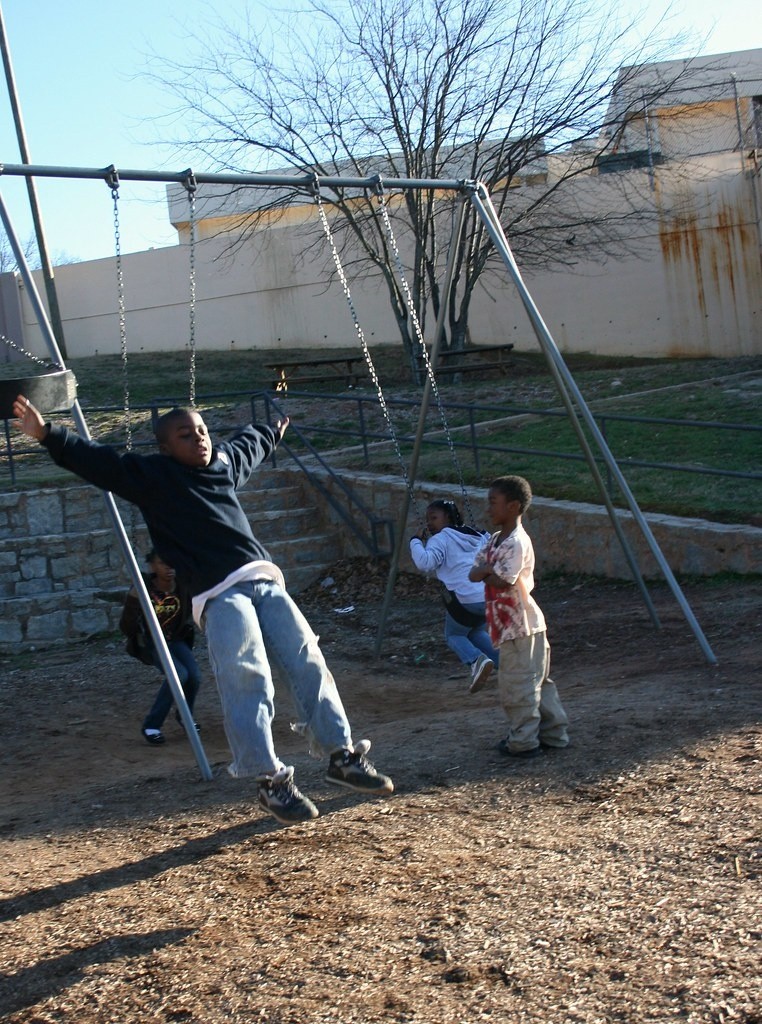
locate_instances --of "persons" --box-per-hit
[409,498,500,693]
[11,396,393,825]
[468,477,570,756]
[120,551,200,746]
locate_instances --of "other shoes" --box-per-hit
[486,740,545,763]
[175,715,201,731]
[141,727,166,744]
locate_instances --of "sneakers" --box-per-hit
[325,739,394,793]
[254,761,319,823]
[469,654,494,692]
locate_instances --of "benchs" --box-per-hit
[263,370,367,397]
[415,360,515,386]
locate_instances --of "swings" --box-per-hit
[315,191,490,627]
[105,180,198,669]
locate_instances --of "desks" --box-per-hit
[415,343,514,382]
[263,355,363,396]
